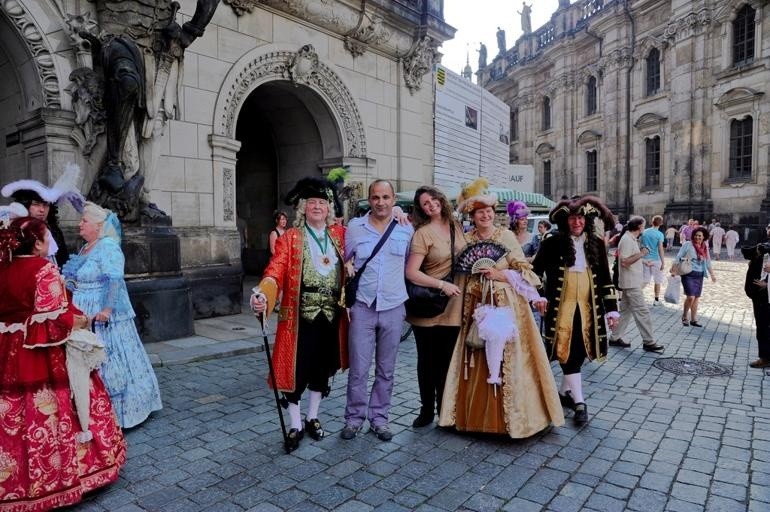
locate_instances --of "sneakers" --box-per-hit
[370,423,392,440]
[749,359,770,367]
[341,422,362,439]
[653,300,662,306]
[413,415,434,427]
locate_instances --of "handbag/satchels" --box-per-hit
[345,272,360,308]
[404,273,455,318]
[664,275,681,303]
[465,302,485,349]
[670,250,692,275]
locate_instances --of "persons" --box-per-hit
[405,185,470,427]
[532,195,621,423]
[516,1,533,33]
[340,179,414,441]
[0,166,85,269]
[496,27,506,54]
[249,177,409,450]
[604,215,739,351]
[0,201,128,512]
[753,253,770,306]
[475,42,487,69]
[741,221,770,368]
[269,210,288,254]
[62,201,164,428]
[507,201,552,263]
[80,1,222,227]
[437,191,565,439]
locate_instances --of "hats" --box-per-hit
[550,195,615,231]
[457,178,497,214]
[298,179,336,202]
[2,163,80,203]
[507,201,530,218]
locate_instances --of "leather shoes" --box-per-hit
[558,390,576,409]
[574,403,588,423]
[305,417,324,439]
[682,315,689,325]
[643,343,664,350]
[609,339,630,347]
[690,321,702,327]
[285,428,303,450]
